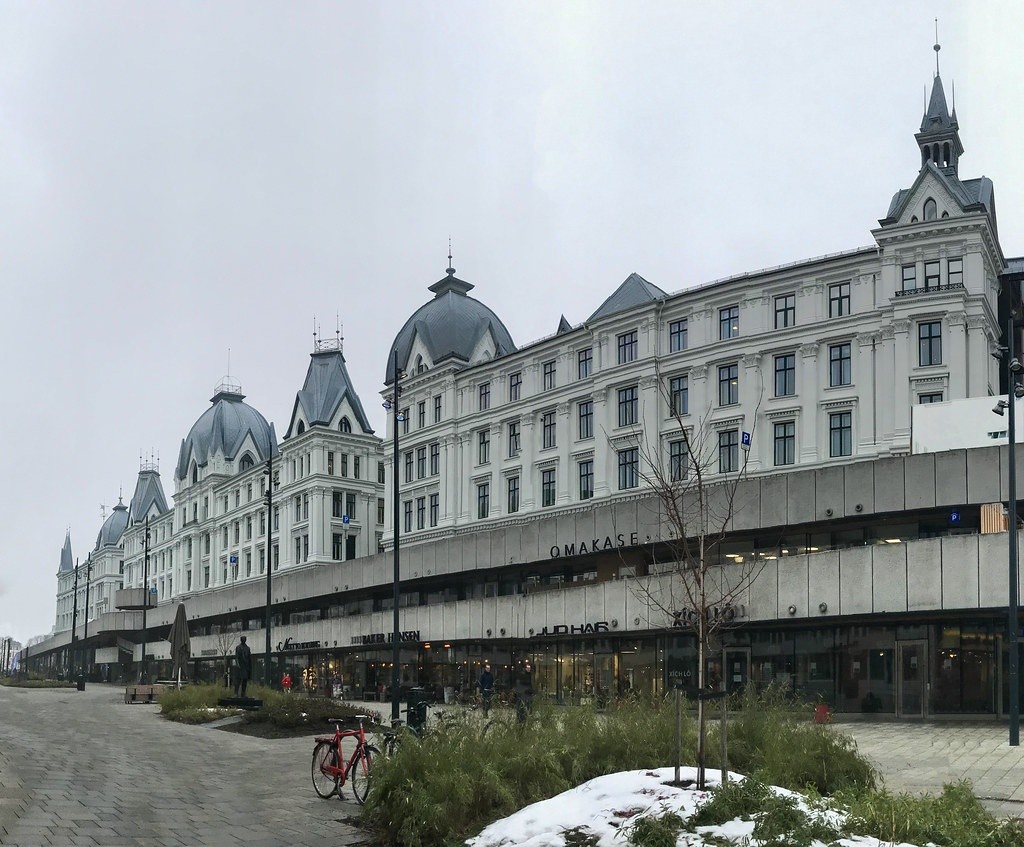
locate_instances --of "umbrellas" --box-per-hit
[167,602,192,680]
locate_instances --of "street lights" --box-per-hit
[382,350,409,732]
[69,557,81,683]
[992,320,1024,746]
[139,515,151,686]
[77,552,93,690]
[263,442,281,685]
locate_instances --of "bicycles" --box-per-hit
[313,716,392,806]
[384,702,514,760]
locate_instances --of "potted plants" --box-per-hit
[815,689,829,724]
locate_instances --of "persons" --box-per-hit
[309,666,313,687]
[282,673,292,694]
[231,635,252,699]
[312,671,317,693]
[514,664,533,719]
[303,665,308,693]
[479,664,494,718]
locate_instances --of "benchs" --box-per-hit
[124,685,167,703]
